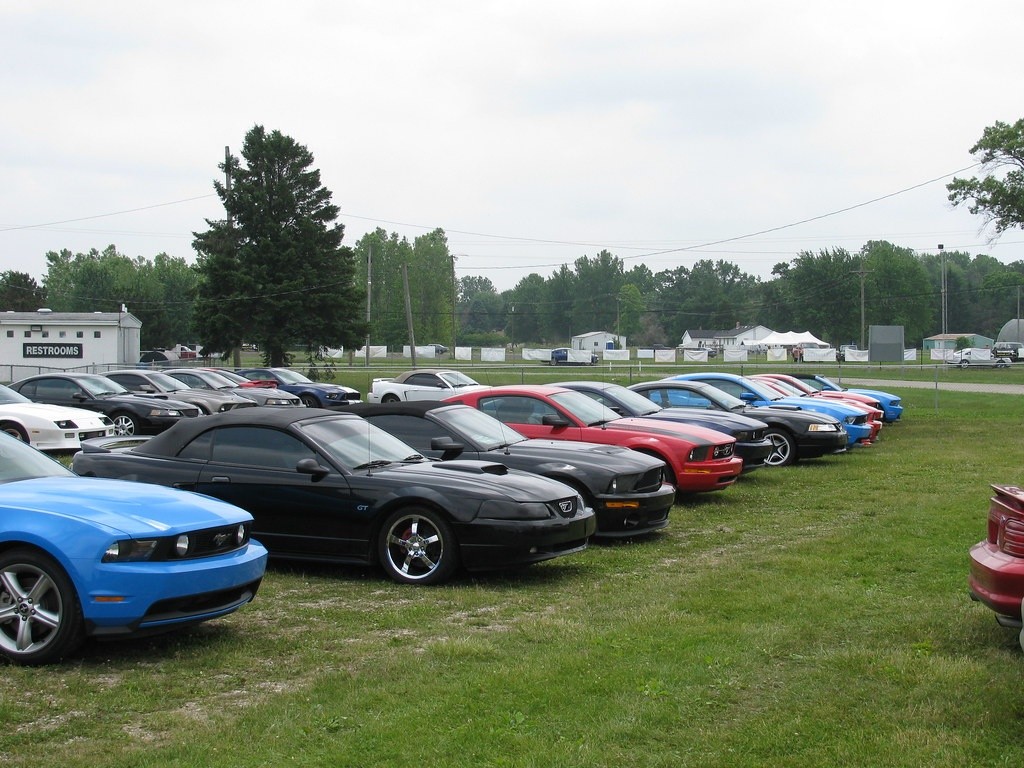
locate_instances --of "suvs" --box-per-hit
[992,342,1024,359]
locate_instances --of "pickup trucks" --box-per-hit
[836,344,858,361]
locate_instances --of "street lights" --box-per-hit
[938,244,946,372]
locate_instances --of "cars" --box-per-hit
[426,343,450,354]
[541,348,599,366]
[0,431,269,665]
[700,347,717,359]
[968,481,1024,653]
[140,351,169,369]
[791,341,823,363]
[947,347,1013,369]
[0,368,904,589]
[171,346,196,358]
